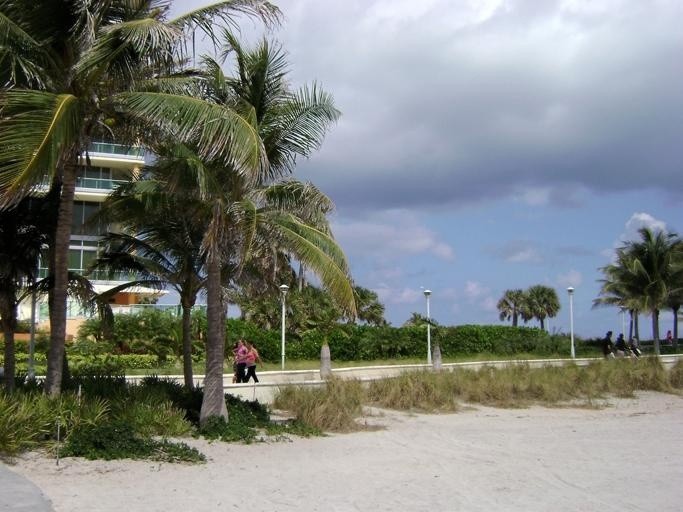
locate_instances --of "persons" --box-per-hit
[239,340,264,382]
[234,338,246,383]
[231,341,239,383]
[602,330,614,361]
[614,331,626,358]
[666,330,672,345]
[628,336,640,364]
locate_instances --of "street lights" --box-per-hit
[423,289,433,363]
[567,286,576,359]
[277,284,291,370]
